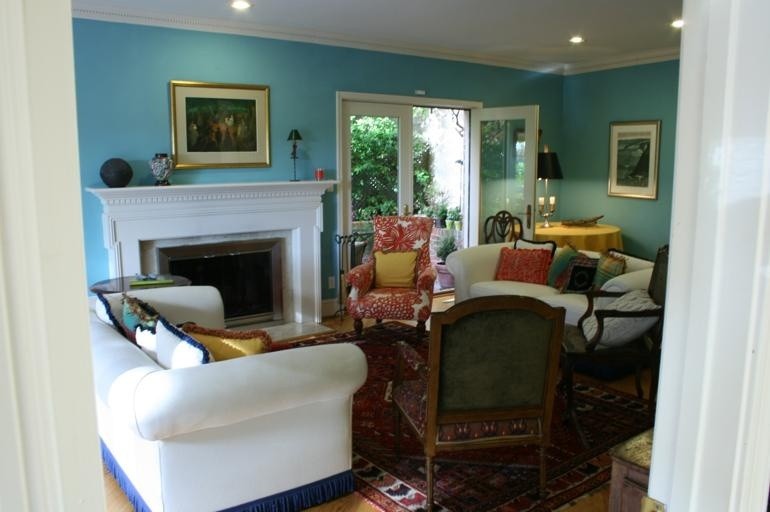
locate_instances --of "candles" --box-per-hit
[538,196,557,206]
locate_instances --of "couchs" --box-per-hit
[86,275,368,511]
[444,242,660,355]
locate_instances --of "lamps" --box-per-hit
[533,152,564,196]
[286,126,304,182]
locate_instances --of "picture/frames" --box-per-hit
[607,119,662,200]
[168,79,272,170]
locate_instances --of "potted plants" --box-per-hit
[435,237,460,288]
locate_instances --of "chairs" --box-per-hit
[386,294,567,512]
[345,212,437,340]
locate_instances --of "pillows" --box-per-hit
[493,238,626,293]
[374,252,418,288]
[95,291,271,365]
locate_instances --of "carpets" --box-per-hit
[270,317,653,511]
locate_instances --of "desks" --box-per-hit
[509,221,622,253]
[91,273,194,294]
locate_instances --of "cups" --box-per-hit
[315,168,324,180]
